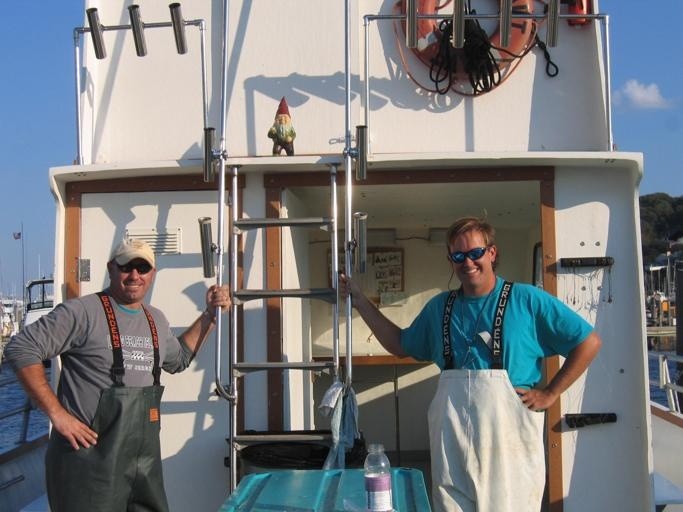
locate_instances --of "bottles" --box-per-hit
[366,441,392,510]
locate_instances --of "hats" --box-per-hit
[110,239,156,269]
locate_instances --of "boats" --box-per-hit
[644,250,676,335]
[21,274,53,329]
[0,296,24,363]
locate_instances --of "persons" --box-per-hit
[267,96,295,156]
[336,215,603,511]
[3,238,230,511]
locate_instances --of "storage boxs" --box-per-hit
[213,465,432,510]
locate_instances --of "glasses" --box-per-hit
[117,263,152,274]
[448,246,488,264]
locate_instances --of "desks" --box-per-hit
[308,355,436,461]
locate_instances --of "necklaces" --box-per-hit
[460,284,493,362]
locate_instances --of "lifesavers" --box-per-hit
[399,0,534,80]
